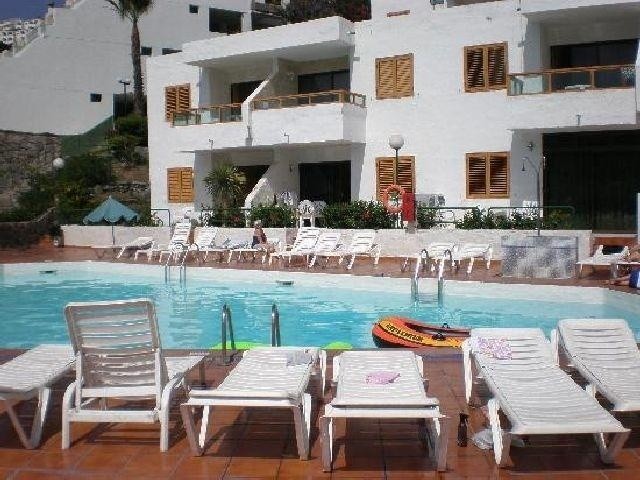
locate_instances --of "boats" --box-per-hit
[372,316,472,349]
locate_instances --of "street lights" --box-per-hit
[51,157,65,182]
[116,76,131,121]
[389,134,404,185]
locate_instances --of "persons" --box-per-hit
[609,242,640,290]
[252,220,269,257]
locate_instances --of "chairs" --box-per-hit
[462,327,630,467]
[179,346,328,460]
[92,220,383,269]
[401,241,493,273]
[576,245,632,280]
[318,350,447,471]
[549,319,640,423]
[1,344,75,449]
[62,298,206,453]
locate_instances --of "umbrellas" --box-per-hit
[84,194,141,250]
